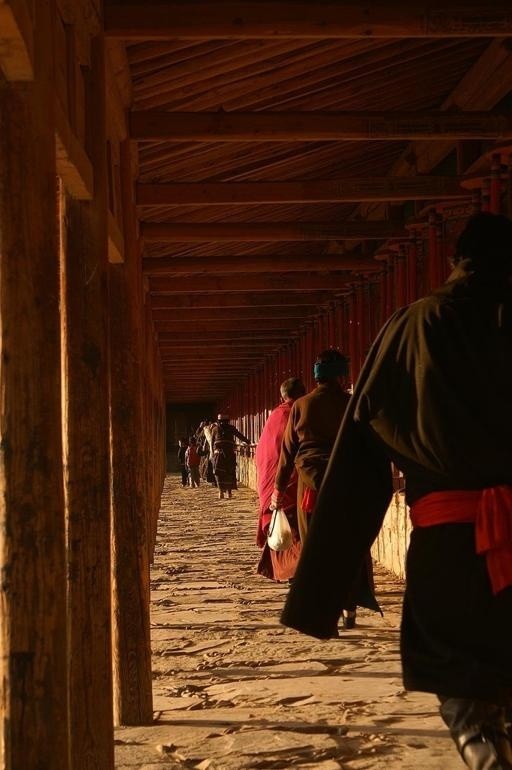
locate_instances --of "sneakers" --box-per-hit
[228,490,231,497]
[341,610,357,629]
[183,481,199,488]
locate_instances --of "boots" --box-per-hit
[220,491,224,498]
[454,722,512,770]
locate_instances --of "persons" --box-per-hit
[254,377,306,586]
[178,414,251,500]
[271,350,384,629]
[280,211,512,770]
